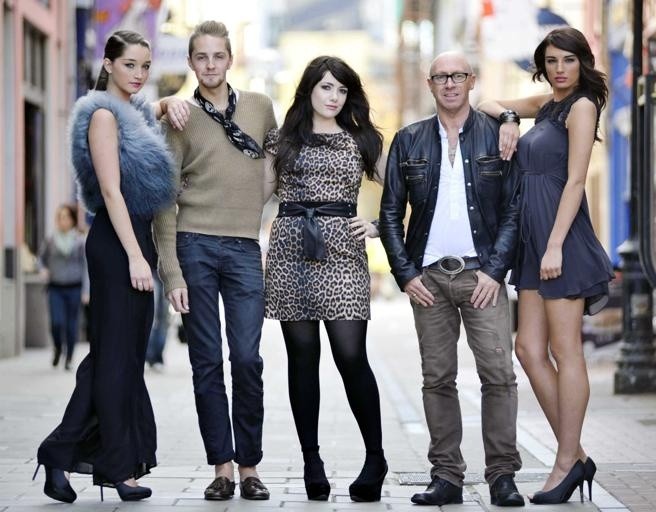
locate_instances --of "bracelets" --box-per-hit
[370,216,383,233]
[496,109,522,126]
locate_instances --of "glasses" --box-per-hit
[429,72,472,87]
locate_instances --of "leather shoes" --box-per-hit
[237,469,271,503]
[202,476,239,505]
[409,476,466,507]
[487,473,526,508]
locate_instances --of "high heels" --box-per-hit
[97,472,153,503]
[30,443,78,505]
[524,455,598,507]
[346,454,390,503]
[299,450,335,503]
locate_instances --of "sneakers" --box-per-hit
[64,358,72,371]
[52,350,62,367]
[152,362,166,375]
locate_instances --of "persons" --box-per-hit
[378,51,524,507]
[262,54,386,501]
[475,26,614,503]
[35,202,91,370]
[30,28,191,505]
[152,19,279,501]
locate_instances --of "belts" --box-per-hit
[421,253,482,278]
